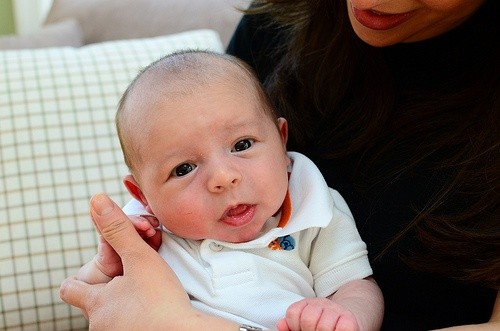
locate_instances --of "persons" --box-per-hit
[75,47,384,331]
[61,0,500,331]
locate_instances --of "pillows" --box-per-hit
[0,28,225,331]
